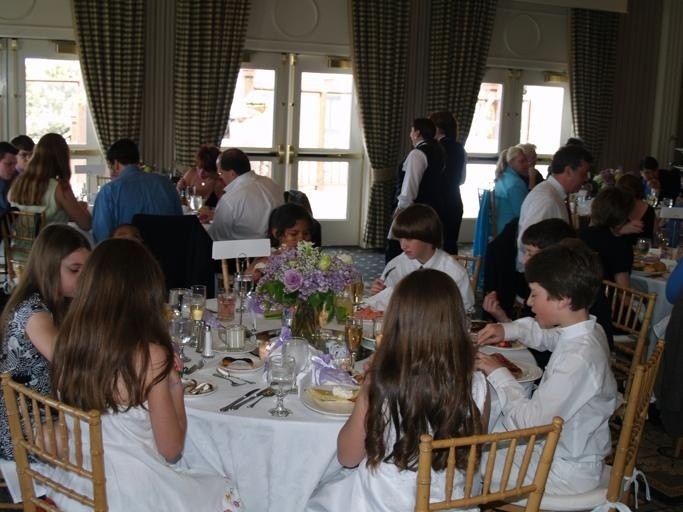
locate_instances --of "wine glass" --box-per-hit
[234,273,254,314]
[185,185,194,210]
[344,272,364,313]
[282,337,309,394]
[661,197,673,208]
[346,316,363,369]
[656,231,670,247]
[267,356,296,417]
[80,182,88,201]
[466,318,472,335]
[164,285,207,363]
[636,238,650,255]
[647,195,657,208]
[578,196,591,209]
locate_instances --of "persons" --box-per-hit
[513,143,593,274]
[0,141,19,239]
[429,109,468,256]
[613,173,661,247]
[472,234,618,498]
[243,201,314,292]
[198,147,287,275]
[514,143,543,190]
[493,145,529,234]
[90,138,183,245]
[1,222,94,507]
[638,154,679,201]
[487,218,615,386]
[175,143,226,206]
[308,266,484,512]
[545,135,585,193]
[369,201,477,318]
[573,186,636,321]
[383,116,448,265]
[656,252,683,437]
[10,134,36,177]
[6,133,93,278]
[110,222,144,243]
[48,235,237,512]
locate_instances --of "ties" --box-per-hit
[564,197,572,225]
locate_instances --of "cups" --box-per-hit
[372,315,384,339]
[218,323,246,352]
[661,258,677,273]
[215,288,237,321]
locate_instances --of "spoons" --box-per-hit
[213,374,247,386]
[216,368,256,384]
[247,390,277,409]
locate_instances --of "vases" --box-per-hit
[292,299,329,354]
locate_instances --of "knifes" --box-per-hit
[230,388,269,411]
[218,388,260,412]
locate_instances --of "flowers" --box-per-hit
[247,239,361,341]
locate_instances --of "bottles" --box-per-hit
[584,171,593,200]
[568,192,577,214]
[196,322,206,353]
[202,326,214,358]
[190,195,201,215]
[191,321,201,348]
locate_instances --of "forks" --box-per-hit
[381,267,396,284]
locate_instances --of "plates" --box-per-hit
[469,318,491,334]
[204,298,219,317]
[183,384,218,398]
[361,322,376,343]
[213,341,256,354]
[216,355,264,372]
[484,341,527,350]
[632,270,664,277]
[301,384,360,416]
[507,360,542,383]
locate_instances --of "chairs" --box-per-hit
[606,337,663,512]
[212,238,271,296]
[416,417,563,512]
[454,254,480,292]
[477,187,497,237]
[0,371,108,512]
[3,206,43,283]
[602,279,658,387]
